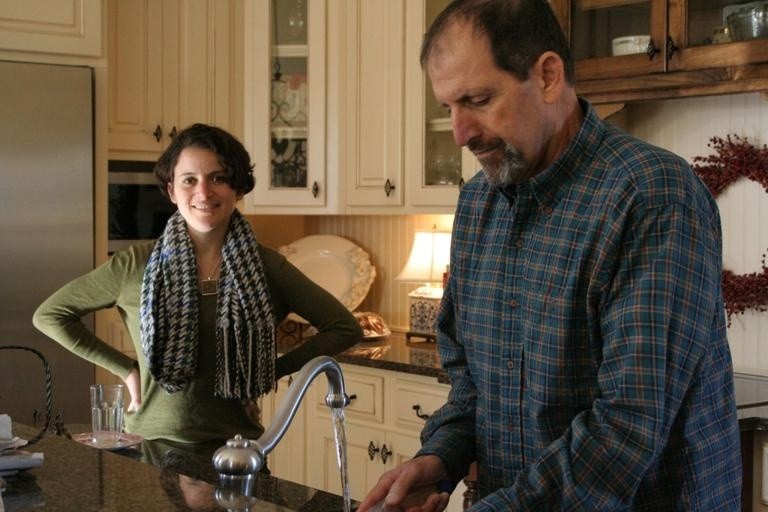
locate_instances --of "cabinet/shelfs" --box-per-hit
[105,0,232,161]
[310,362,464,511]
[242,0,478,216]
[256,372,305,487]
[1,2,104,60]
[550,1,767,105]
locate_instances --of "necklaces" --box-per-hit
[196,252,225,282]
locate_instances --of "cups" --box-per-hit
[90,384,126,448]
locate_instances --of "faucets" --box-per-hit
[210,353,349,494]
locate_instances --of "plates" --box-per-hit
[70,431,143,451]
[276,234,378,326]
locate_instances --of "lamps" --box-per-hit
[394,230,451,345]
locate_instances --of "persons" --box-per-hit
[158,466,251,512]
[340,0,745,512]
[27,121,365,481]
